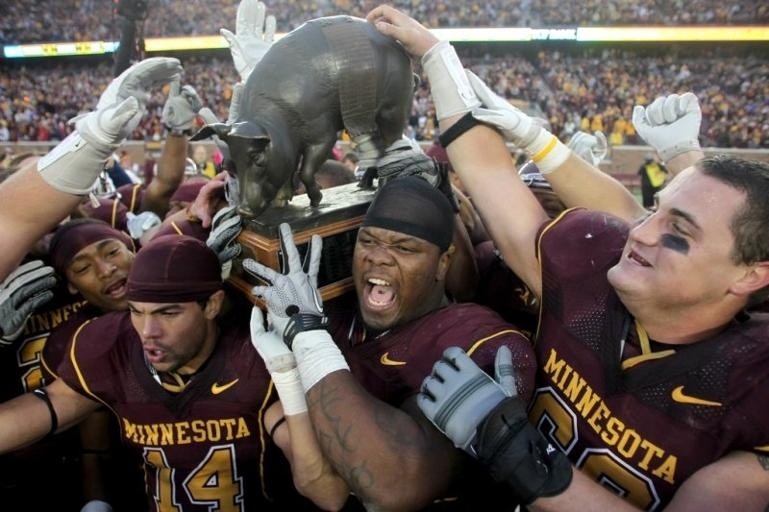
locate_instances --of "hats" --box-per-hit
[47,176,222,303]
[360,175,456,253]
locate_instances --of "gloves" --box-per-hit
[0,260,56,347]
[566,129,609,165]
[38,56,182,198]
[633,92,702,164]
[414,343,519,461]
[243,223,334,372]
[206,206,244,263]
[160,74,204,135]
[463,68,539,151]
[220,0,276,84]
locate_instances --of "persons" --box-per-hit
[0,1,768,512]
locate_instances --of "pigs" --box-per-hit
[187,14,421,218]
